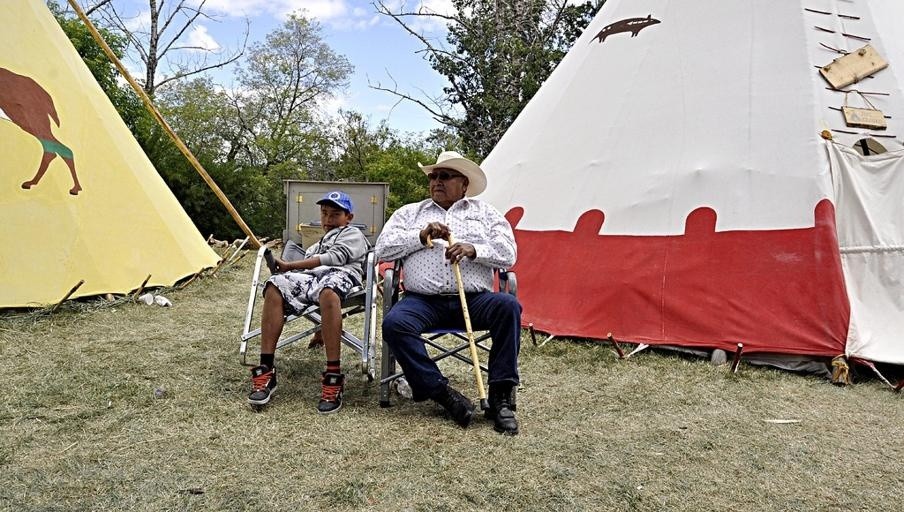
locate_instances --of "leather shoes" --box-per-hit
[444,388,473,428]
[484,404,518,434]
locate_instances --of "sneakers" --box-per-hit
[246,365,277,404]
[317,371,345,415]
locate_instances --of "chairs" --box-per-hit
[378,258,522,412]
[238,238,375,406]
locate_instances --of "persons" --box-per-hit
[246,190,370,416]
[374,151,522,436]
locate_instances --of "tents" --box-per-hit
[1,0,225,310]
[378,0,904,392]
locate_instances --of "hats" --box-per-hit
[315,191,353,214]
[417,151,487,197]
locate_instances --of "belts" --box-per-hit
[426,293,471,301]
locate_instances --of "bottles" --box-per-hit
[391,375,413,400]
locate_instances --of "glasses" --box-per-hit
[428,171,463,179]
